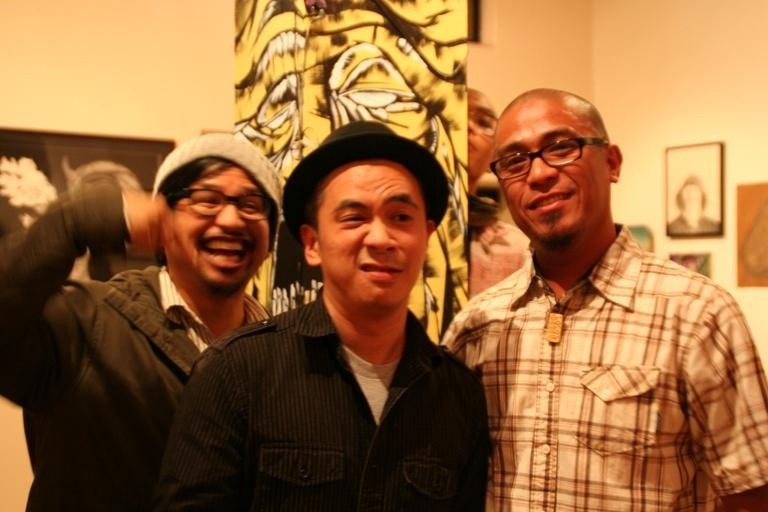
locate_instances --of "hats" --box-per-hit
[152,133,281,218]
[282,121,448,247]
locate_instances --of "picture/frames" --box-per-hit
[0,128,175,280]
[665,143,723,236]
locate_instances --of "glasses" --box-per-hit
[166,187,274,221]
[490,137,607,181]
[468,111,498,137]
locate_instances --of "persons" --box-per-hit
[455,86,533,304]
[1,129,283,512]
[439,87,768,512]
[668,175,720,233]
[156,122,491,512]
